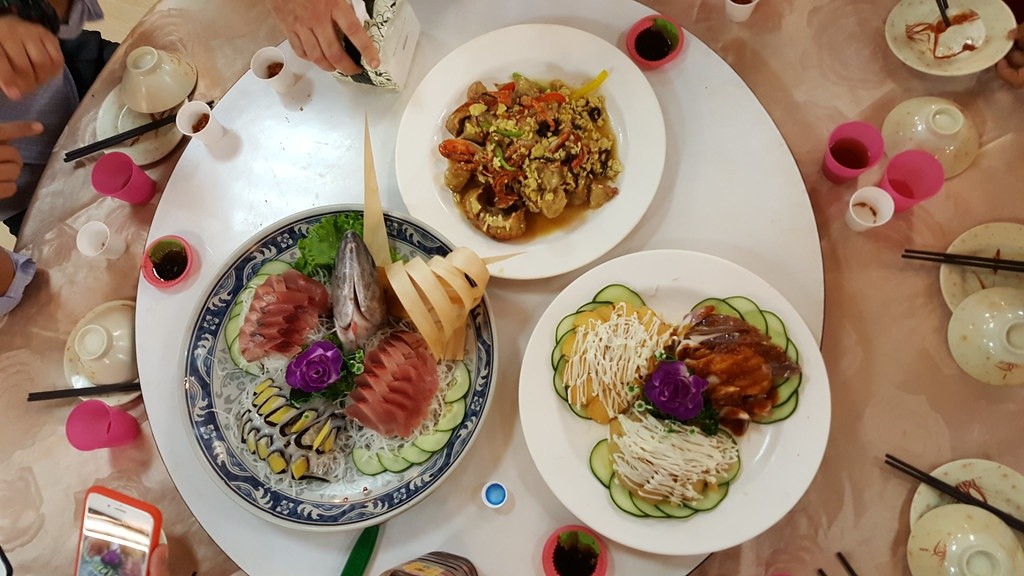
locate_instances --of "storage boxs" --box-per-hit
[332,0,421,90]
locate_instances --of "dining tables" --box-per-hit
[0,0,1024,576]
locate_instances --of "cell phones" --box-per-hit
[74,485,161,576]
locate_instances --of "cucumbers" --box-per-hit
[223,260,294,378]
[552,284,803,519]
[351,361,471,475]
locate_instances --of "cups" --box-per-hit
[845,186,895,231]
[822,121,885,185]
[176,101,224,144]
[250,46,296,92]
[724,0,761,23]
[90,152,158,207]
[66,399,141,452]
[878,149,945,214]
[76,221,127,260]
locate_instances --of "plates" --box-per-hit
[395,24,667,281]
[62,300,142,406]
[96,82,189,165]
[910,459,1024,549]
[519,248,833,555]
[177,205,499,533]
[940,222,1024,312]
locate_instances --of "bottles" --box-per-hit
[379,551,479,576]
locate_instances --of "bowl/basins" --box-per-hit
[947,287,1024,386]
[908,504,1024,576]
[142,234,194,288]
[885,0,1017,76]
[74,307,138,384]
[120,45,197,114]
[541,526,608,576]
[626,15,685,67]
[881,96,979,179]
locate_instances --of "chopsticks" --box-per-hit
[63,100,215,164]
[813,550,859,576]
[884,451,1024,532]
[901,248,1024,273]
[935,0,950,27]
[26,383,141,402]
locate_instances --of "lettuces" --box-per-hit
[291,212,406,285]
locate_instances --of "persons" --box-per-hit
[266,0,380,76]
[0,0,121,319]
